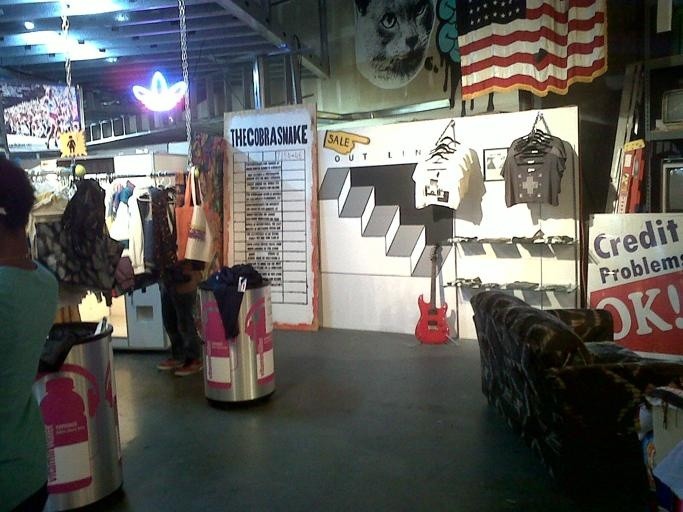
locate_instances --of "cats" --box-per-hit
[352,0,434,88]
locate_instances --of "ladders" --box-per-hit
[604,62,643,216]
[616,139,644,214]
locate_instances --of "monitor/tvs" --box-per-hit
[662,89,683,129]
[0,77,87,161]
[660,156,683,213]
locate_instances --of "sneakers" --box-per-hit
[175,358,205,377]
[156,354,181,372]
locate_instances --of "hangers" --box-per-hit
[418,119,467,174]
[92,166,201,200]
[21,166,73,199]
[508,111,564,166]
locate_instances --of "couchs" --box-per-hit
[468,291,679,481]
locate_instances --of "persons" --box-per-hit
[67,136,76,152]
[154,258,204,375]
[0,159,57,512]
[4,86,80,149]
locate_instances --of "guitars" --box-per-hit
[415,245,450,344]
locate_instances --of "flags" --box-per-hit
[455,1,609,100]
